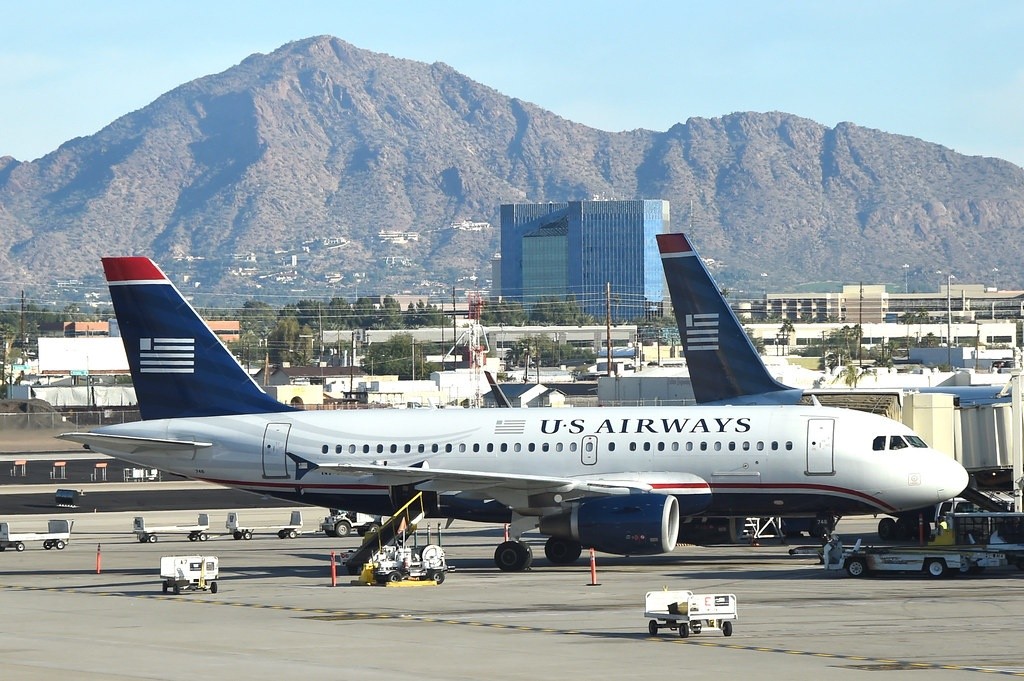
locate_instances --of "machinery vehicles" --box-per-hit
[321,509,383,537]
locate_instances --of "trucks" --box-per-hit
[822,497,1024,583]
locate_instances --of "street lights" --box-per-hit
[946,274,956,369]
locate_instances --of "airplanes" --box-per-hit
[54,231,969,571]
